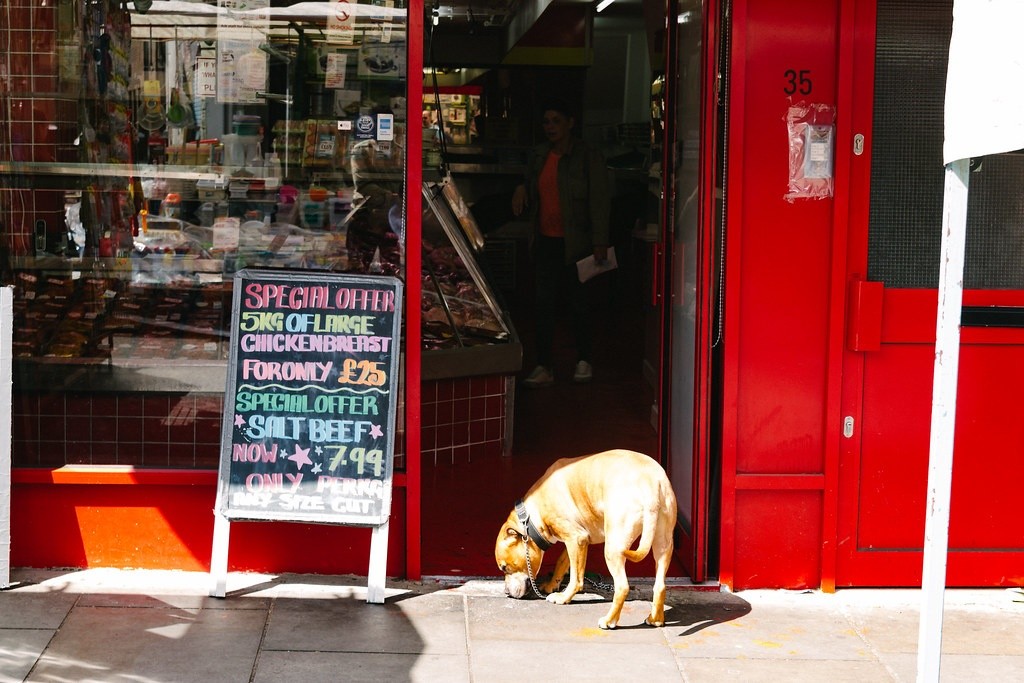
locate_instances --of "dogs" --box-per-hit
[495,448,679,630]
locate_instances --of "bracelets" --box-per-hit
[392,193,398,200]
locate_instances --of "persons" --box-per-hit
[511,99,610,386]
[345,106,404,276]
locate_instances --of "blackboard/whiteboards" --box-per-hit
[215,268,405,523]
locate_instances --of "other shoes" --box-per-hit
[574,360,594,379]
[524,366,554,383]
